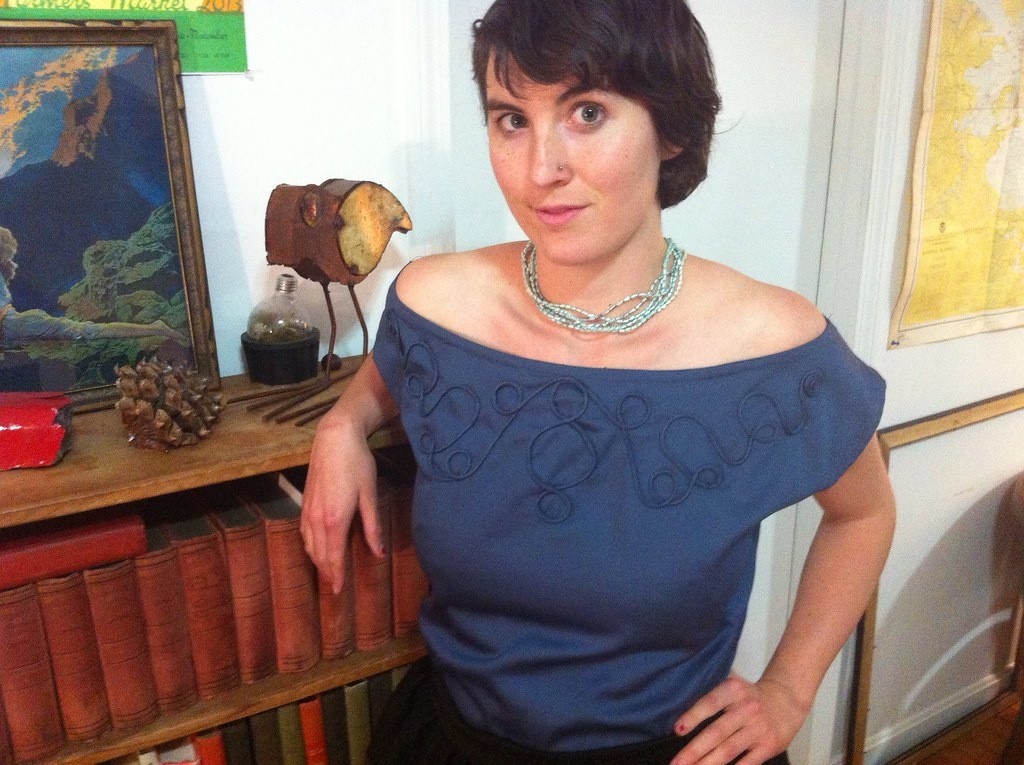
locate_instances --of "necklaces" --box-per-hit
[522,238,687,333]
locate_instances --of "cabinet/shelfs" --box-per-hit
[0,357,431,765]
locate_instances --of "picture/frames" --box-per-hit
[850,393,1024,765]
[0,17,222,416]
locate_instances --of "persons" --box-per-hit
[301,0,897,765]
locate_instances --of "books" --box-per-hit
[1,446,432,765]
